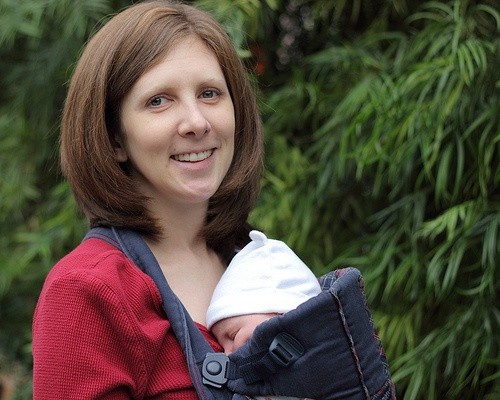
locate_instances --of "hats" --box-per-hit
[205,230,322,331]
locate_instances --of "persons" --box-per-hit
[204,229,322,356]
[34,0,267,400]
